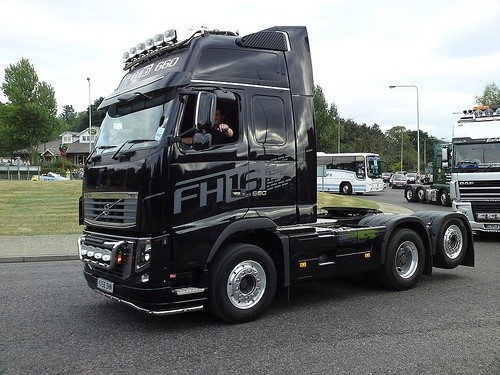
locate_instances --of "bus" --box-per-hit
[317,151,389,196]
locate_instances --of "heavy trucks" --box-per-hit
[78,24,477,323]
[451,105,500,236]
[403,140,452,206]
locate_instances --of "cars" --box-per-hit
[395,171,419,184]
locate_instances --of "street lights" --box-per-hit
[388,84,420,184]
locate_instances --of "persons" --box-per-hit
[181,106,236,150]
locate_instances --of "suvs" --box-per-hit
[390,174,405,186]
[392,175,407,189]
[382,172,394,183]
